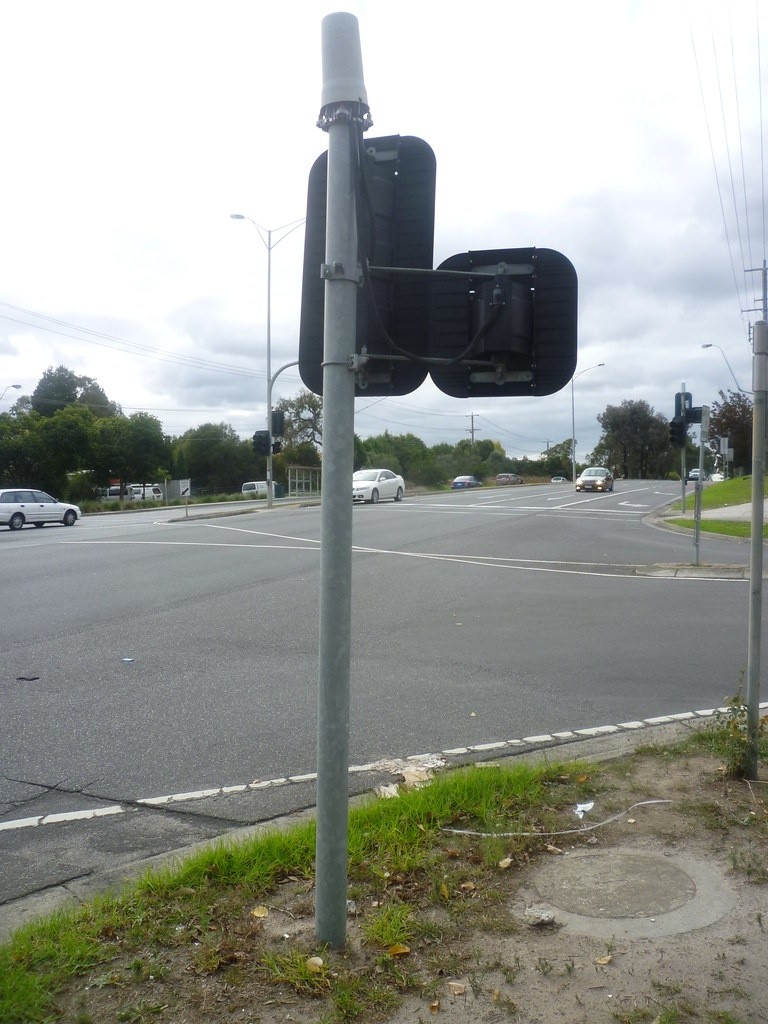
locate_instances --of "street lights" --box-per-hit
[573,363,605,482]
[231,213,307,505]
[701,344,756,394]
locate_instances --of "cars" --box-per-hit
[451,476,484,489]
[352,469,405,503]
[551,476,568,483]
[576,468,614,493]
[496,473,524,484]
[689,468,705,478]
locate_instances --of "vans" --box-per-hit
[95,485,133,506]
[242,480,278,498]
[128,484,165,502]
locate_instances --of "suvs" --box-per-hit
[0,489,81,530]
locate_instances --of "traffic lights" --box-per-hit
[670,416,686,448]
[252,430,271,456]
[273,442,280,455]
